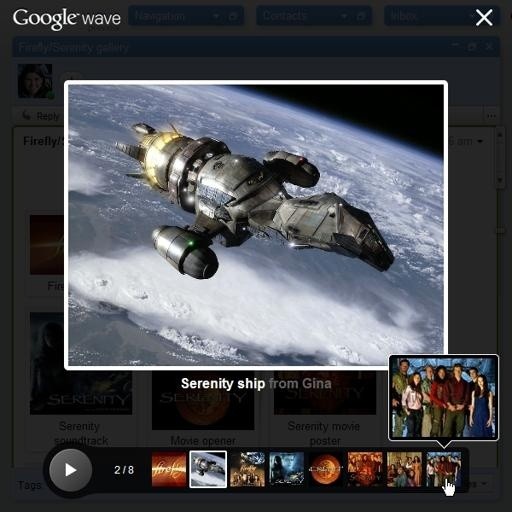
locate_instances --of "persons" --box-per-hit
[427,453,463,487]
[391,359,493,437]
[349,452,384,487]
[388,456,423,487]
